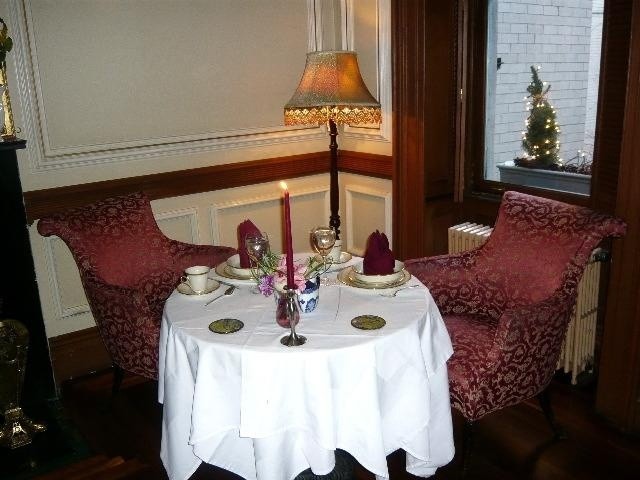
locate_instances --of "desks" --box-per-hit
[160,249,452,478]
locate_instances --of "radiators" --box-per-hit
[449,219,606,388]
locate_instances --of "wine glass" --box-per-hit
[311,226,337,288]
[245,231,271,295]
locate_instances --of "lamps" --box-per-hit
[281,46,382,261]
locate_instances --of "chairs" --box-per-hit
[406,191,624,428]
[38,190,239,399]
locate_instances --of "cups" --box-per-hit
[180,266,210,294]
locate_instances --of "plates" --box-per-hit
[214,253,273,280]
[177,278,220,296]
[336,260,411,289]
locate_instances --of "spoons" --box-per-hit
[204,285,235,307]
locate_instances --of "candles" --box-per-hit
[280,179,295,291]
